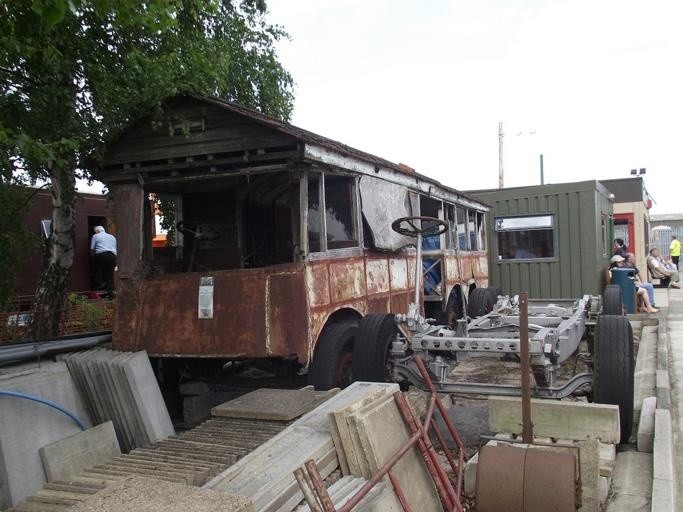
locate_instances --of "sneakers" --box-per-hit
[670,285,680,289]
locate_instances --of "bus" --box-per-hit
[85,94,501,391]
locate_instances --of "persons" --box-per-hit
[610,232,681,311]
[89,224,116,294]
[509,244,542,260]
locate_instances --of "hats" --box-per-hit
[611,255,625,262]
